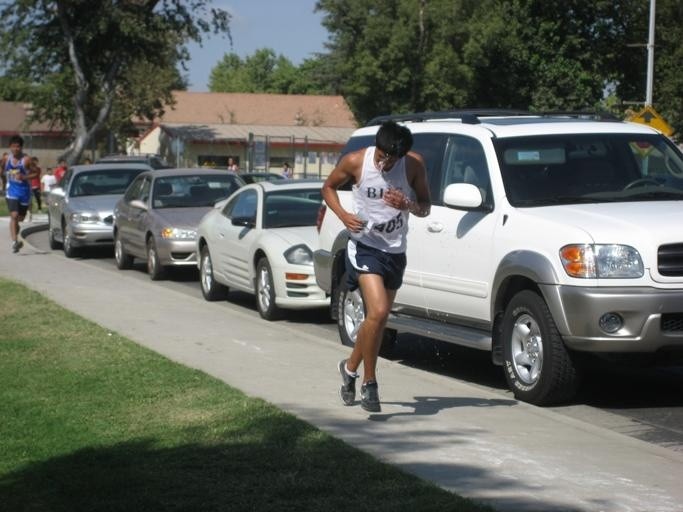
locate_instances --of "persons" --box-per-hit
[0,152,92,222]
[0,134,38,253]
[282,162,289,177]
[321,119,431,412]
[227,157,239,175]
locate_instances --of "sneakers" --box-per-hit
[359,381,381,413]
[11,241,23,254]
[337,358,359,406]
